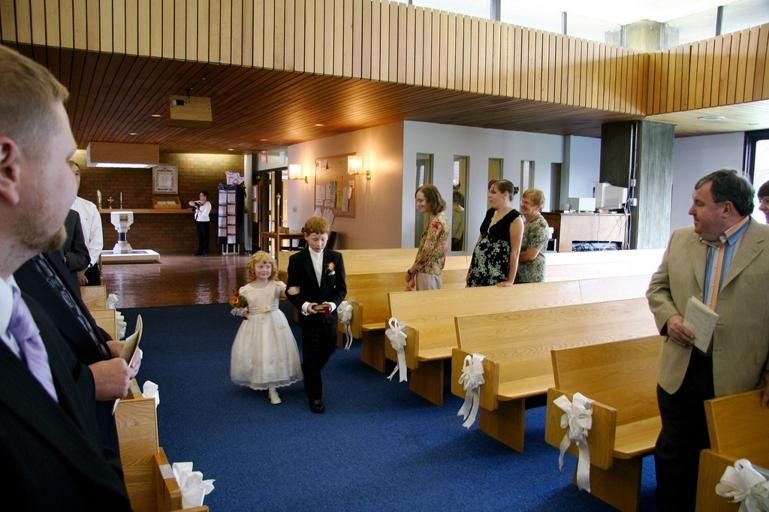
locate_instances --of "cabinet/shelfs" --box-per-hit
[216,187,244,254]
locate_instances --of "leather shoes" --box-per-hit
[309,399,325,413]
[267,390,282,405]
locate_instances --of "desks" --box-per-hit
[534,212,630,252]
[259,231,306,269]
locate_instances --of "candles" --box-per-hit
[119,191,122,202]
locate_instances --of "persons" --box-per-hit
[285,215,347,413]
[465,179,524,288]
[189,190,211,256]
[646,170,769,512]
[404,184,450,291]
[757,180,769,224]
[451,179,465,251]
[513,188,549,284]
[230,251,304,405]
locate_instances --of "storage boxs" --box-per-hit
[566,196,597,214]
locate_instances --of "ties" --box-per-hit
[36,254,110,356]
[314,253,322,288]
[8,288,60,407]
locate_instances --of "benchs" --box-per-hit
[275,246,655,407]
[297,228,339,250]
[452,245,768,511]
[76,283,215,511]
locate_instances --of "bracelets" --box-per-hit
[408,269,413,275]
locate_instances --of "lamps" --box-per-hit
[347,154,372,181]
[287,164,308,185]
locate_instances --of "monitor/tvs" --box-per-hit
[595,182,628,209]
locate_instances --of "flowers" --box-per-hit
[227,293,251,319]
